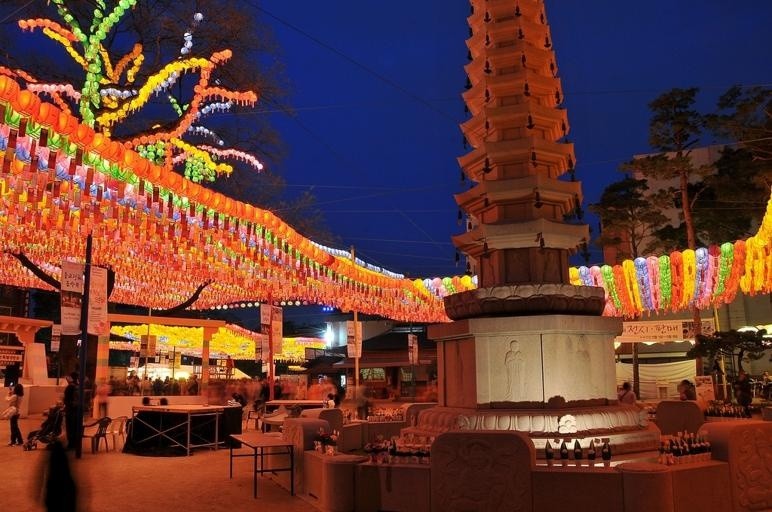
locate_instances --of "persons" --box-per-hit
[2,384,25,445]
[63,372,81,451]
[96,370,338,411]
[732,372,753,418]
[618,381,637,405]
[677,380,696,400]
[225,354,234,379]
[762,371,771,381]
[502,339,529,400]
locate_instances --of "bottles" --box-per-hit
[657,429,711,465]
[368,433,435,465]
[365,406,403,422]
[545,438,553,458]
[315,426,341,456]
[574,439,582,458]
[560,441,567,459]
[602,440,611,459]
[587,441,596,458]
[703,399,745,419]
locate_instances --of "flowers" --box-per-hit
[364,440,392,454]
[314,426,340,446]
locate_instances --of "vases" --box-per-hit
[314,441,336,456]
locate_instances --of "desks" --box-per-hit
[229,434,295,498]
[264,400,328,432]
[133,404,224,456]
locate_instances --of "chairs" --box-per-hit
[245,408,263,430]
[82,416,127,454]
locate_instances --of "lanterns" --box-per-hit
[0,1,478,362]
[569,188,772,320]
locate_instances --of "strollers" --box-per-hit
[22,404,67,452]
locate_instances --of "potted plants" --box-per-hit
[687,329,767,403]
